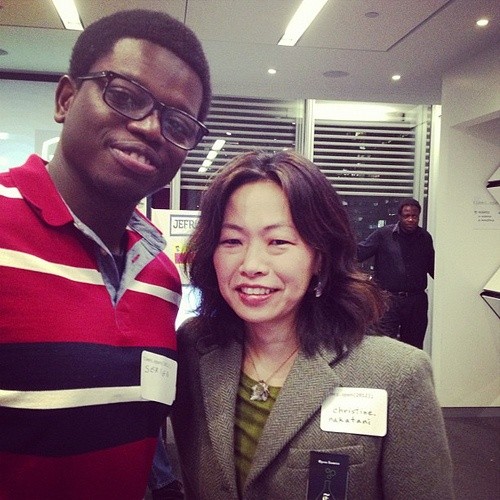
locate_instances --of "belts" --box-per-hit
[386,287,428,298]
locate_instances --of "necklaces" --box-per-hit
[243,341,302,402]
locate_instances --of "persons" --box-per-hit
[168,149,456,500]
[356,197,435,350]
[146,417,186,500]
[1,9,212,500]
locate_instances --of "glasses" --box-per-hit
[76,69,211,152]
